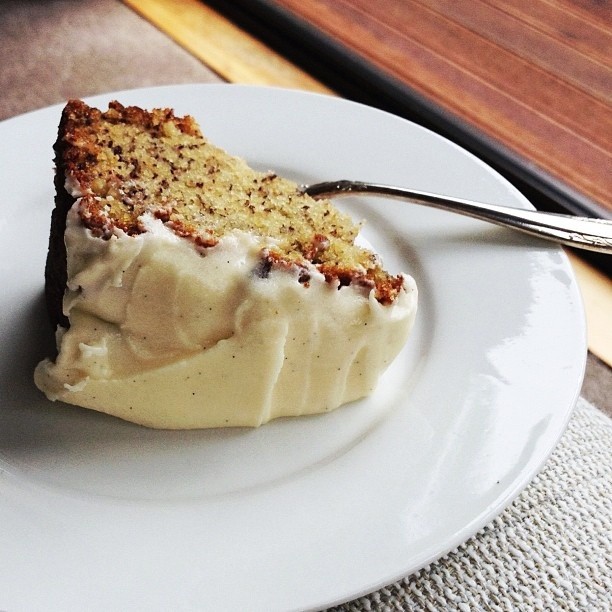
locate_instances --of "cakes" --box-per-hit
[33,98,419,430]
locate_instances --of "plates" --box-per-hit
[0,83,587,611]
